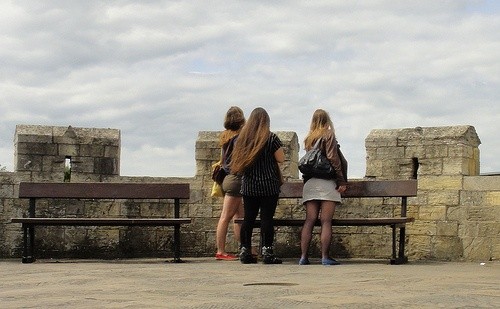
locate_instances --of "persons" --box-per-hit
[240,107,287,264]
[215,106,247,261]
[299,109,348,265]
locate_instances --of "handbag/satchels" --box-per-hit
[297,136,335,179]
[211,181,224,198]
[212,166,225,184]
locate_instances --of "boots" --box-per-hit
[240,246,257,263]
[261,246,282,264]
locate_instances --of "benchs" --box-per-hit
[233,180,417,265]
[10,182,192,264]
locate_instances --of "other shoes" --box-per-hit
[252,248,258,256]
[299,258,310,265]
[215,252,239,261]
[322,257,340,265]
